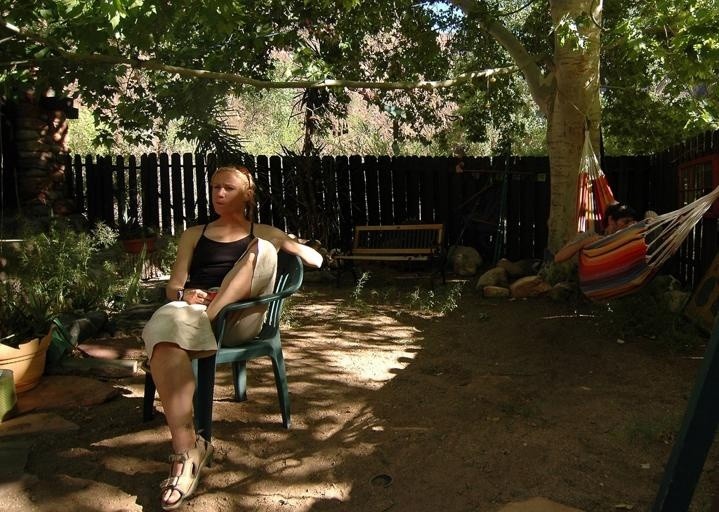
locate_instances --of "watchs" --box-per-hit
[176,287,187,302]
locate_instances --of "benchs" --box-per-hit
[332,224,448,289]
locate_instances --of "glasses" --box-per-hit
[218,161,251,189]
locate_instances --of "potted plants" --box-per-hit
[117,216,155,254]
[0,280,61,395]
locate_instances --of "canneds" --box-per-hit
[207,287,220,302]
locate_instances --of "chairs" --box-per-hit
[140,249,305,442]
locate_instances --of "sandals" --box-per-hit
[159,432,213,510]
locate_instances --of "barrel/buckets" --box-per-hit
[0,369,20,420]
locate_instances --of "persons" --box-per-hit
[136,161,326,509]
[554,198,687,309]
